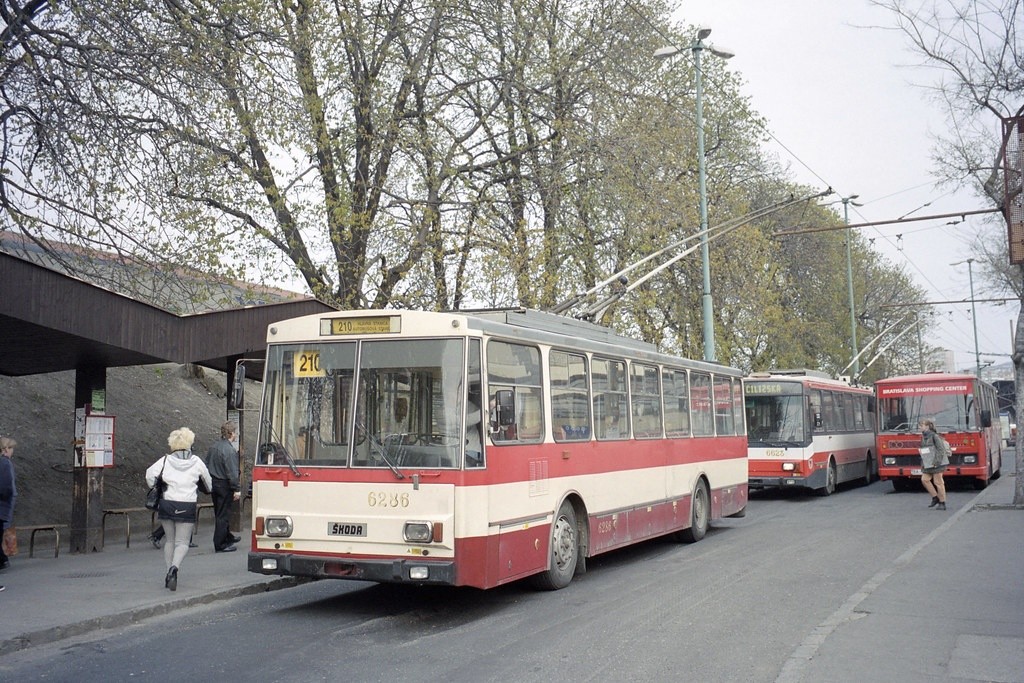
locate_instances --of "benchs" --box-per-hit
[102,508,154,548]
[194,503,214,535]
[14,523,69,560]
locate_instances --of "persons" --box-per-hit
[0,437,18,593]
[919,419,950,510]
[205,421,242,553]
[145,427,212,591]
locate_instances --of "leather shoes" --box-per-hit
[216,546,237,553]
[232,536,241,542]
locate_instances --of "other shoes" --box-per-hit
[929,496,939,507]
[937,502,947,510]
[0,561,10,570]
[0,585,5,591]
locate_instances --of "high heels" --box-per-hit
[189,543,198,547]
[165,566,178,591]
[147,534,160,549]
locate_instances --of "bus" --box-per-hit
[875,361,1002,491]
[741,305,926,496]
[229,187,835,593]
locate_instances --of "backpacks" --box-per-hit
[922,433,952,457]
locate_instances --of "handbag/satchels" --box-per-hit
[2,527,17,556]
[145,454,167,510]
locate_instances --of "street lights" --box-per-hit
[949,258,987,379]
[651,25,737,364]
[842,194,860,385]
